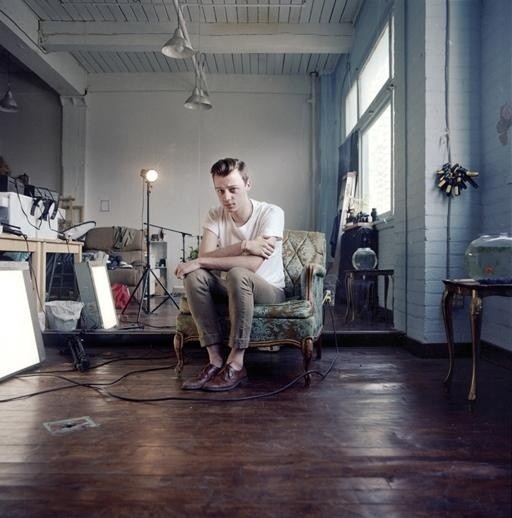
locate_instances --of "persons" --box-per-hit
[174,157,287,393]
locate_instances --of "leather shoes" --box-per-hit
[181,364,247,391]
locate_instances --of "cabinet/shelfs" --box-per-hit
[149,241,169,297]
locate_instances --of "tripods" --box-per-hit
[68,334,90,371]
[122,187,181,321]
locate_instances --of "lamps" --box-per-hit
[121,168,179,324]
[160,8,213,111]
[0,54,20,112]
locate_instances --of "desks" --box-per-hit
[0,235,85,312]
[343,269,393,327]
[440,278,511,414]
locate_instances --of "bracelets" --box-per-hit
[241,238,249,253]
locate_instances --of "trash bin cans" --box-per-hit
[45,300,83,331]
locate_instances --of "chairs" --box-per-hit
[172,229,328,388]
[84,225,145,304]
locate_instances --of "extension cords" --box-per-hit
[323,282,336,308]
